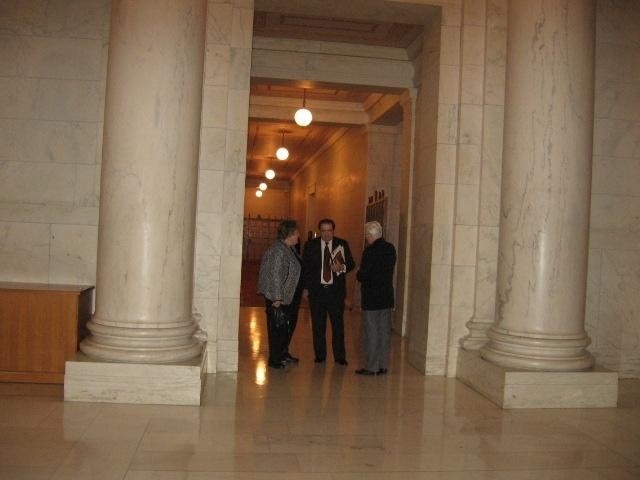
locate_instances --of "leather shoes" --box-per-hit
[267,355,300,368]
[353,366,387,375]
[314,356,328,363]
[336,355,348,365]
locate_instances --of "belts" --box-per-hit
[319,284,334,289]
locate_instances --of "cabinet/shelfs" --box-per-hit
[0,282,94,383]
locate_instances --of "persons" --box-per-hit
[258,218,396,374]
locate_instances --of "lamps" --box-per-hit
[264,158,275,180]
[294,88,312,128]
[276,128,289,160]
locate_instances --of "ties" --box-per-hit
[323,241,332,283]
